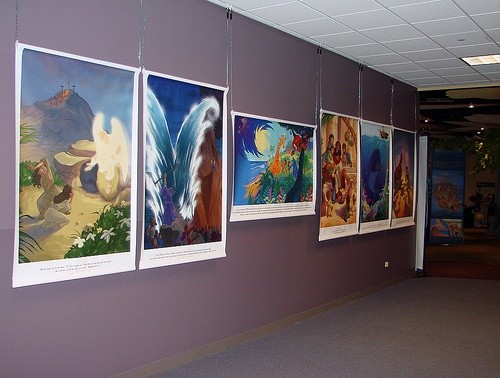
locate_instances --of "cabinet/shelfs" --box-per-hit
[427,168,465,244]
